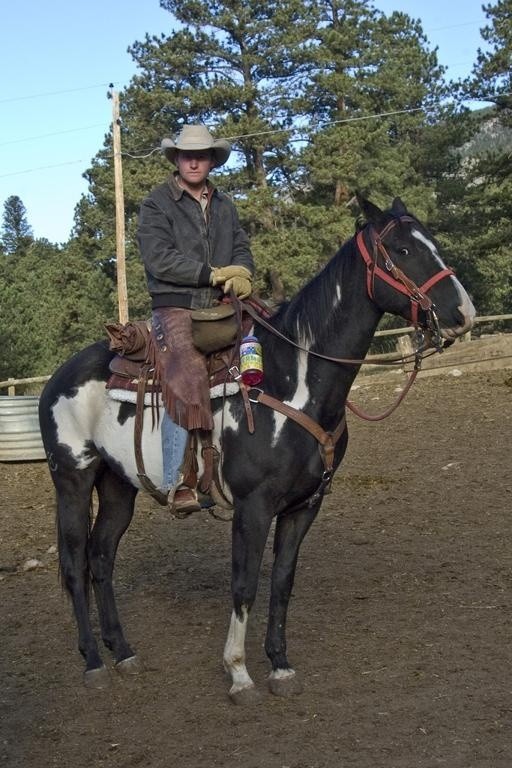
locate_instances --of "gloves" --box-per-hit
[223,275,252,301]
[207,265,254,289]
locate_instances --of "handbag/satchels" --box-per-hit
[190,304,240,353]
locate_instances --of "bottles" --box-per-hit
[239,336,264,386]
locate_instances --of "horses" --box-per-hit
[33,186,478,710]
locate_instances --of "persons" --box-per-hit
[134,123,256,513]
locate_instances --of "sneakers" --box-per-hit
[168,489,201,513]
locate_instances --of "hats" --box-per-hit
[160,124,230,171]
